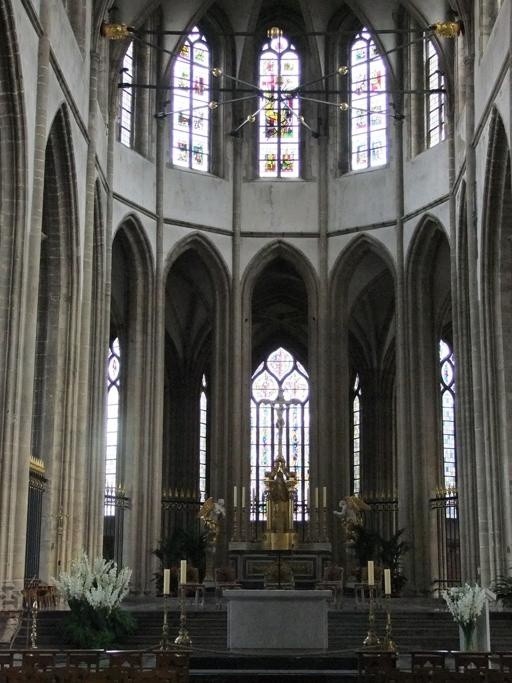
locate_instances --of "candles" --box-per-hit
[178,557,188,584]
[366,558,376,585]
[305,484,328,508]
[382,566,392,594]
[233,484,257,507]
[163,567,171,593]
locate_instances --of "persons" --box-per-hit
[333,499,358,537]
[206,498,227,534]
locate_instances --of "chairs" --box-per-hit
[176,558,382,608]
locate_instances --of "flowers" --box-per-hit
[434,579,493,651]
[48,551,140,650]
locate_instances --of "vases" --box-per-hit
[458,617,478,653]
